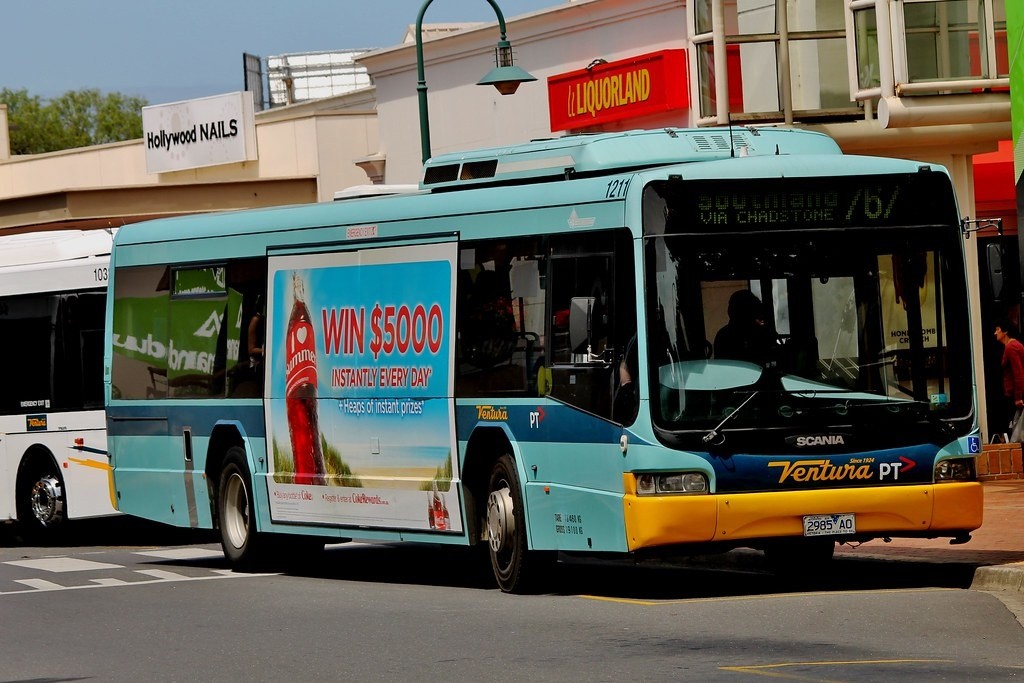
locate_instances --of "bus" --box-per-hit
[103,126,1021,597]
[0,185,432,547]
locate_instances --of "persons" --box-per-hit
[247,309,264,379]
[620,289,778,426]
[994,319,1024,443]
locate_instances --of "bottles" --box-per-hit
[441,494,451,530]
[285,271,328,487]
[433,480,446,530]
[427,491,435,529]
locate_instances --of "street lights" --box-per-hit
[415,0,539,169]
